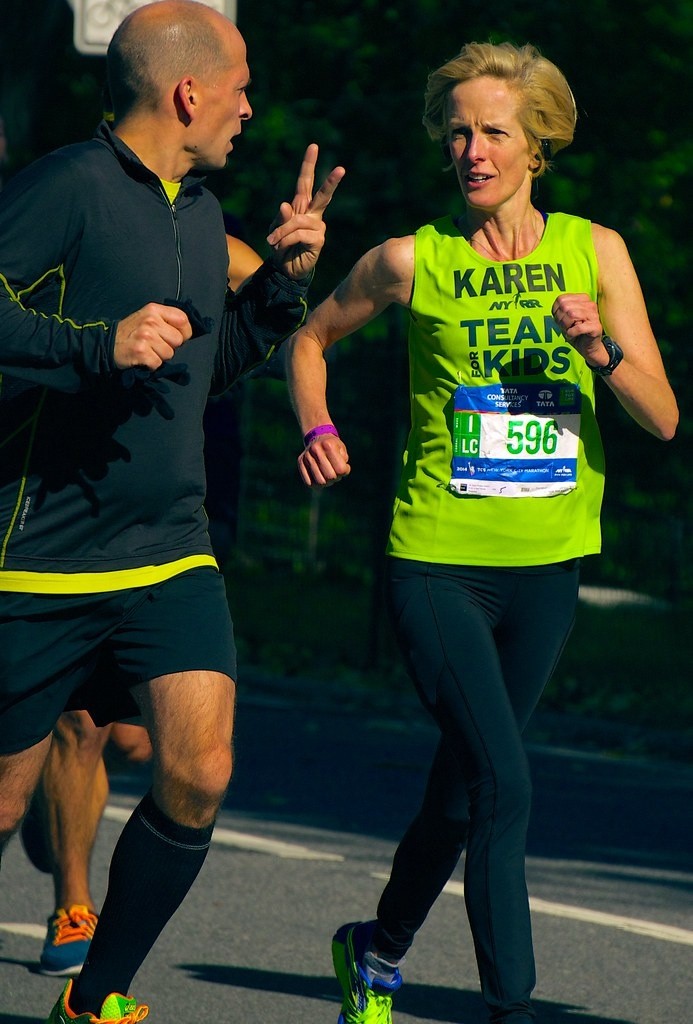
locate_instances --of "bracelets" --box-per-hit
[585,336,624,375]
[304,425,338,446]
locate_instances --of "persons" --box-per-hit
[0,1,345,1024]
[285,42,679,1024]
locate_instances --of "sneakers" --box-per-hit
[19,793,55,874]
[330,921,404,1024]
[47,978,151,1024]
[37,904,99,977]
